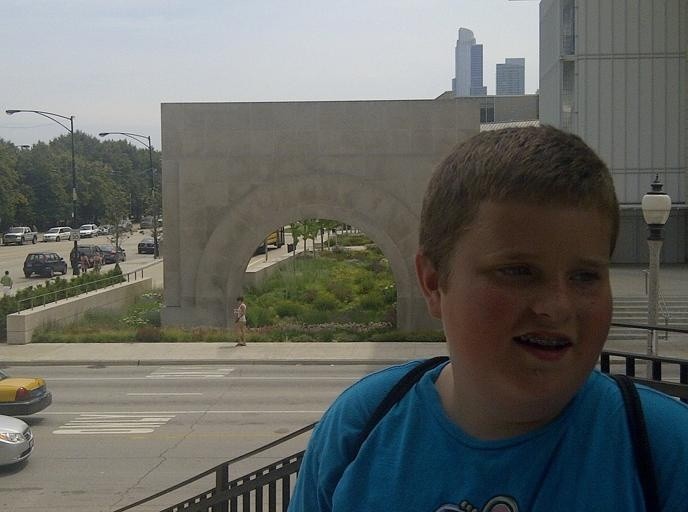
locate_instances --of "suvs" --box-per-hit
[21,252,71,278]
[69,244,106,267]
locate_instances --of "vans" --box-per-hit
[44,224,76,243]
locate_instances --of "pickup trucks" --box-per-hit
[2,225,41,245]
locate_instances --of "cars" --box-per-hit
[100,245,127,263]
[79,215,163,255]
[0,364,59,418]
[1,411,35,466]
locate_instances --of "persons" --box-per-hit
[80,253,90,273]
[287,123,688,511]
[94,252,101,272]
[233,297,248,346]
[0,270,13,297]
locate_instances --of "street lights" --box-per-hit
[5,108,83,251]
[632,177,676,380]
[97,128,161,261]
